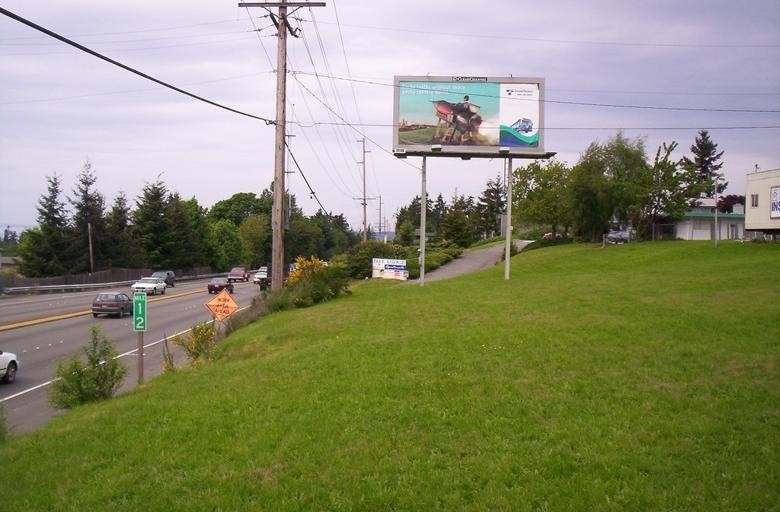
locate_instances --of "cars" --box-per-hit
[206,277,234,294]
[130,276,166,296]
[91,292,135,319]
[227,261,333,285]
[0,350,21,385]
[151,270,176,288]
